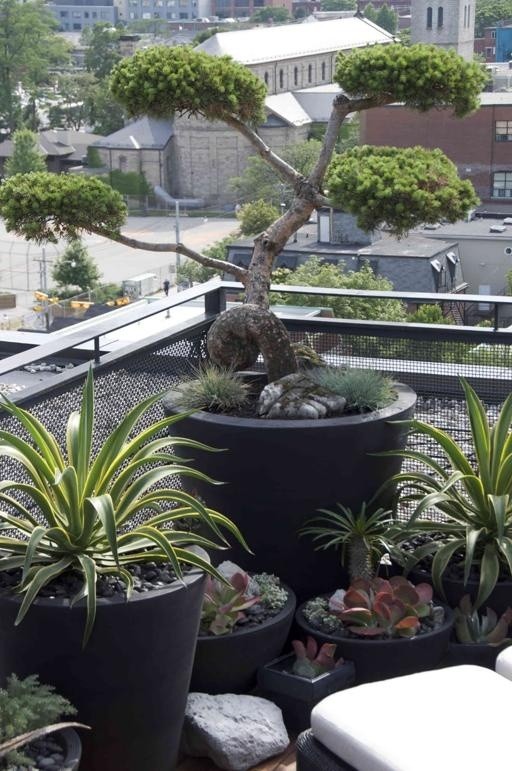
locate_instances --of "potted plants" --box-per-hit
[297,368,512,681]
[261,636,357,720]
[195,565,296,698]
[1,364,254,771]
[2,40,495,590]
